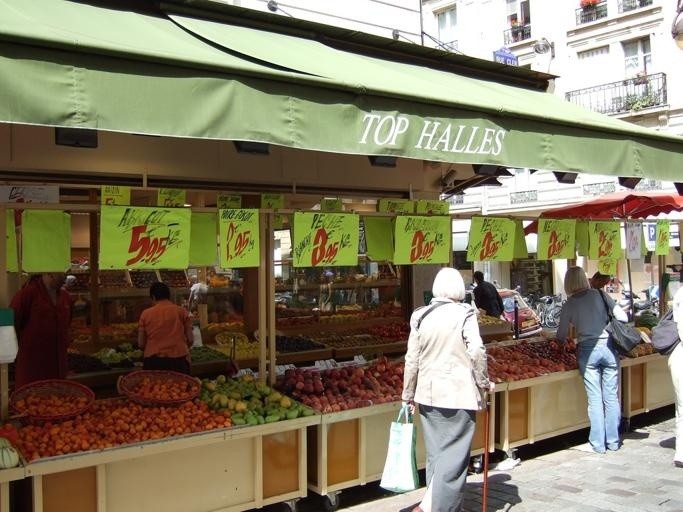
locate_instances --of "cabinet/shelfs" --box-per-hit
[277,267,513,496]
[480,327,676,452]
[0,270,321,512]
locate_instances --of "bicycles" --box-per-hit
[522,290,567,328]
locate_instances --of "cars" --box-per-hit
[494,287,543,339]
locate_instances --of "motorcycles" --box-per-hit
[617,279,658,323]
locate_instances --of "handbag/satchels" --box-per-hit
[651,308,681,356]
[605,316,642,353]
[380,403,421,492]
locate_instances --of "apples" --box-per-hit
[485,337,577,382]
[276,356,405,414]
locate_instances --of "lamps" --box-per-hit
[534,37,555,58]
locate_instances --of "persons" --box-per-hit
[346,265,370,305]
[650,285,659,306]
[10,266,74,390]
[472,270,505,320]
[322,266,335,305]
[493,279,500,288]
[668,288,683,467]
[188,275,208,311]
[559,267,628,454]
[138,283,193,376]
[406,267,495,512]
[589,271,610,289]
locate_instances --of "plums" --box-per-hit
[5,377,231,462]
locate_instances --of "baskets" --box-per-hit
[9,379,96,425]
[118,369,202,407]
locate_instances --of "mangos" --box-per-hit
[200,372,313,425]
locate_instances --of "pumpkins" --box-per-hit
[0,438,13,448]
[0,447,19,468]
